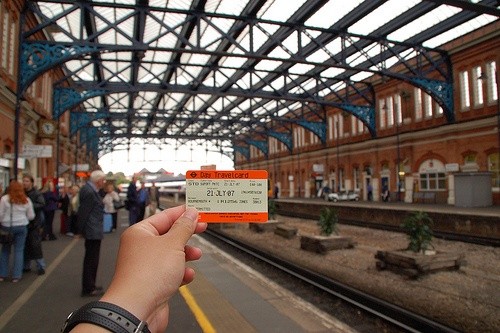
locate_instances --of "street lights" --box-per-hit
[381,92,402,202]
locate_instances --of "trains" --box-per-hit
[119,180,186,199]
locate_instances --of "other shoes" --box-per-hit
[38,269,44,275]
[0,277,5,281]
[27,268,32,272]
[95,286,103,290]
[12,278,19,282]
[83,290,102,296]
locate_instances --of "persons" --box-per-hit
[274,186,279,198]
[368,182,374,200]
[22,175,46,275]
[100,173,159,232]
[37,175,88,241]
[323,183,330,202]
[0,180,36,284]
[382,183,391,200]
[78,169,107,296]
[66,204,208,333]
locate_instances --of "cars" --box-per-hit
[328,189,362,200]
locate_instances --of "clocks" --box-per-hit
[38,118,58,138]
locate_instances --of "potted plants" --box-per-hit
[402,212,441,255]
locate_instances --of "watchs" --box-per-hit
[61,301,151,333]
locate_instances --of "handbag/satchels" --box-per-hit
[0,230,13,245]
[123,200,131,209]
